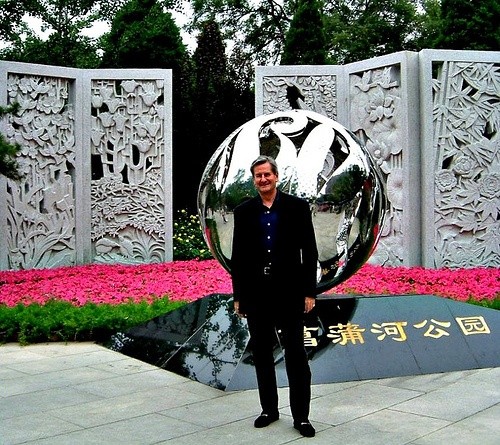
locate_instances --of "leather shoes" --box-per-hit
[294,420,316,438]
[254,411,279,428]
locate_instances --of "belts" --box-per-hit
[256,266,281,275]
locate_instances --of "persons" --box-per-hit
[225,157,318,436]
[220,207,226,222]
